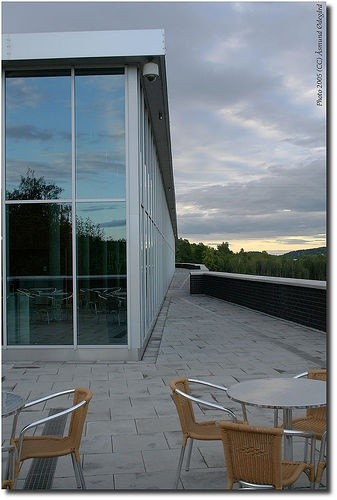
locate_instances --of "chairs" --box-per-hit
[274,369,328,490]
[166,375,248,489]
[217,419,318,490]
[3,387,94,491]
[15,286,127,328]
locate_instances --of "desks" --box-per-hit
[2,391,26,491]
[227,377,327,489]
